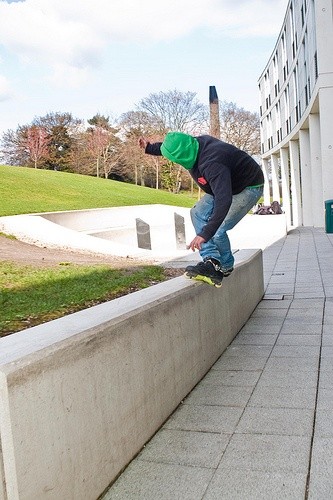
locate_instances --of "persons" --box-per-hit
[138,132,265,288]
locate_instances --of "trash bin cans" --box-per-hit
[324,199,333,234]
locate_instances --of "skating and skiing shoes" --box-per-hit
[184,258,224,288]
[220,265,234,277]
